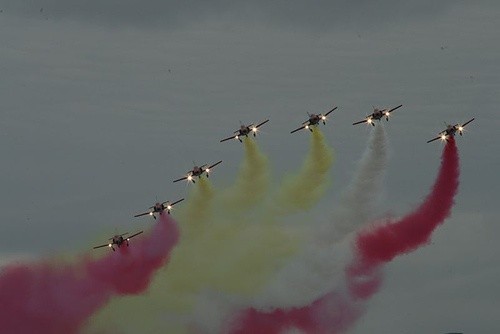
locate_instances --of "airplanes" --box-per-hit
[93,229,145,251]
[221,119,271,144]
[133,198,185,218]
[172,161,223,185]
[426,116,476,143]
[351,103,403,128]
[290,107,339,136]
